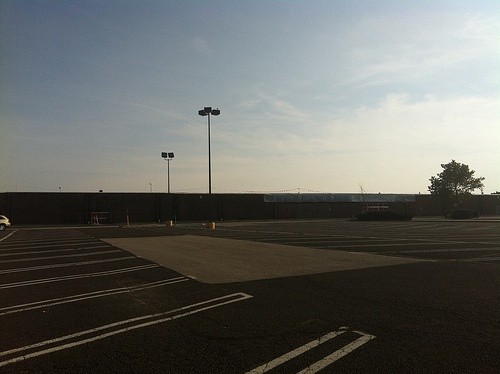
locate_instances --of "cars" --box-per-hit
[0,215,10,232]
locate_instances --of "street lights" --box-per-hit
[198,106,220,231]
[161,152,175,225]
